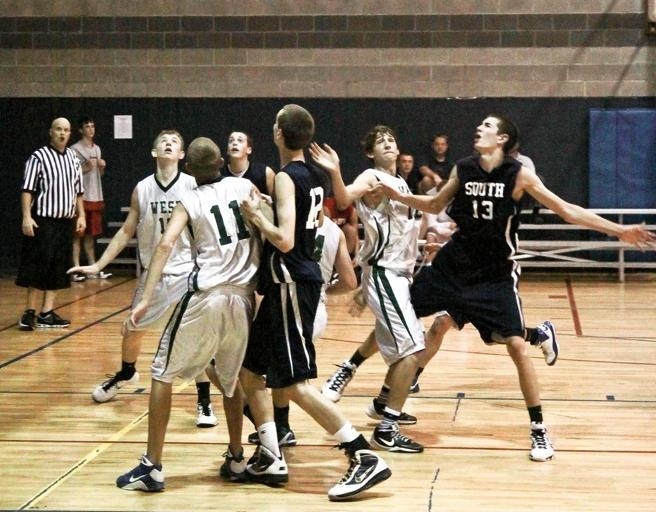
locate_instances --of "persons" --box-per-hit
[309,125,424,451]
[71,117,114,282]
[411,309,558,394]
[320,191,360,286]
[240,104,395,500]
[220,129,275,207]
[509,139,545,225]
[419,131,457,193]
[205,213,360,447]
[396,150,420,219]
[114,136,272,490]
[14,116,87,328]
[425,178,457,263]
[67,127,221,427]
[365,114,655,461]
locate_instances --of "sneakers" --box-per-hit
[18,308,36,330]
[35,310,71,328]
[196,400,219,428]
[91,367,140,404]
[115,454,167,493]
[527,419,556,462]
[320,359,361,404]
[327,449,393,502]
[364,397,418,425]
[246,441,290,485]
[71,271,114,283]
[369,422,425,454]
[537,320,560,366]
[219,445,247,483]
[247,428,297,446]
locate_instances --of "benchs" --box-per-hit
[95,201,656,284]
